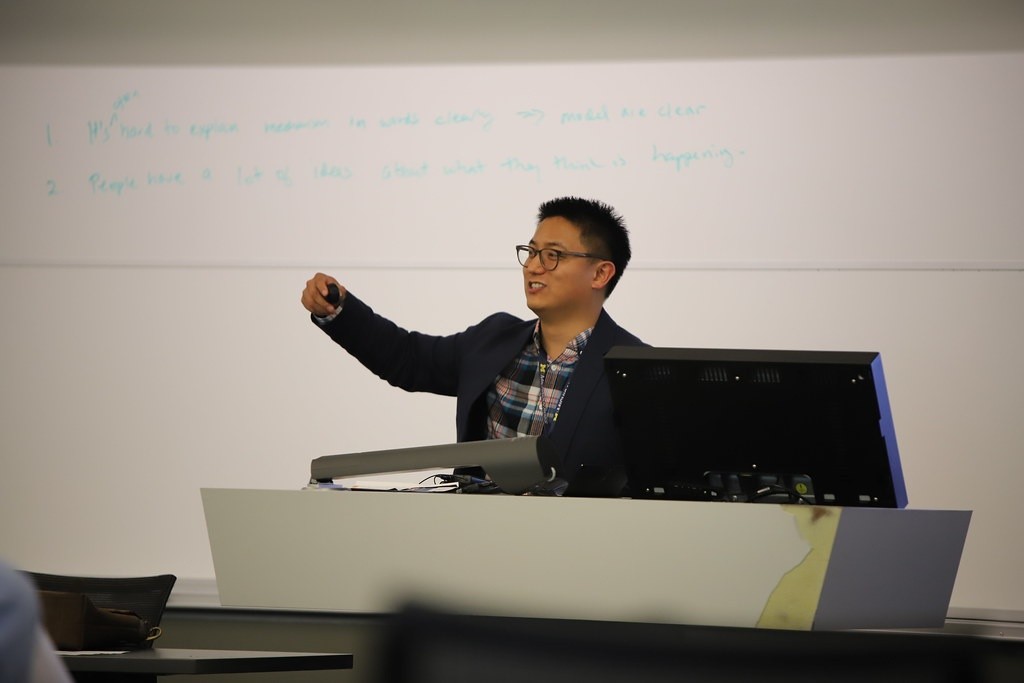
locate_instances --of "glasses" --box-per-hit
[514,244,610,272]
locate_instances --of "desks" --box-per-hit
[53,644,357,673]
[155,577,1023,683]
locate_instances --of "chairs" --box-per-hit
[9,570,178,653]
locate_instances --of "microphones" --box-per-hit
[439,473,490,487]
[454,480,504,494]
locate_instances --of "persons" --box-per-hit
[301,195,656,496]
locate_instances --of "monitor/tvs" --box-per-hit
[604,347,910,508]
